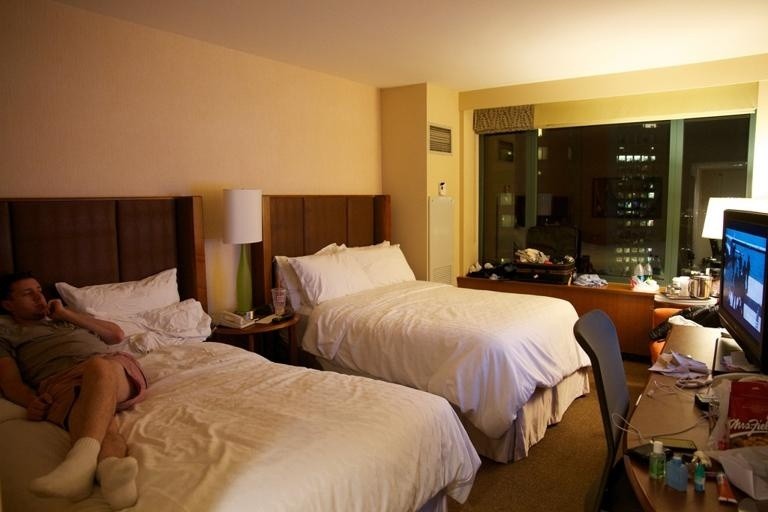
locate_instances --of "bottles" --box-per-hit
[633,260,653,283]
[648,440,688,493]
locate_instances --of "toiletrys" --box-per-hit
[716,469,738,504]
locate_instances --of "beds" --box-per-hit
[262,195,592,465]
[1,195,486,511]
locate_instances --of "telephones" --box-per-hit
[213,311,258,329]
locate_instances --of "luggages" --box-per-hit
[513,224,582,284]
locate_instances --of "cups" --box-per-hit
[687,274,714,300]
[271,288,288,317]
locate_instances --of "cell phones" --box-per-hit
[653,436,697,452]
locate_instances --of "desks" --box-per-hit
[621,327,768,512]
[456,276,655,357]
[654,295,719,309]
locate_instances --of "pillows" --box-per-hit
[55,267,183,315]
[271,239,416,313]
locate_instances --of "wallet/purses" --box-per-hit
[625,442,673,465]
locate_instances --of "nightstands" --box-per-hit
[211,311,302,365]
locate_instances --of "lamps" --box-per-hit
[222,188,264,314]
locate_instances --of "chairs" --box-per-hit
[649,307,683,366]
[573,310,632,453]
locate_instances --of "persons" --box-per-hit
[0,273,149,511]
[722,237,750,316]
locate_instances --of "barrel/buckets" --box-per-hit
[645,261,653,280]
[634,261,645,282]
[689,275,712,299]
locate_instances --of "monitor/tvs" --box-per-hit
[718,209,767,376]
